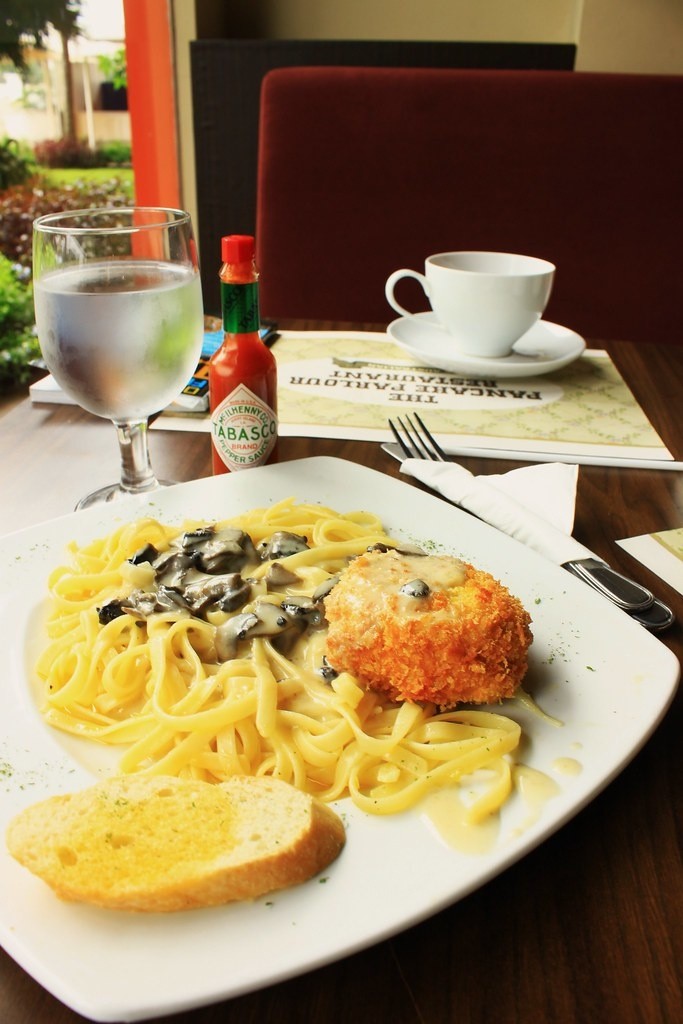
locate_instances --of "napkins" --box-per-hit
[399,457,613,576]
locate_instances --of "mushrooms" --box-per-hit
[91,525,430,682]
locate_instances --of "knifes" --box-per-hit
[381,443,674,629]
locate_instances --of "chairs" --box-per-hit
[252,64,682,349]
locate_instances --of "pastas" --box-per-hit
[31,499,562,825]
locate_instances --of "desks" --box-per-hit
[1,316,682,1024]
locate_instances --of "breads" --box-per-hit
[4,775,345,914]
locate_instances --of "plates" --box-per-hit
[388,313,585,378]
[0,455,680,1022]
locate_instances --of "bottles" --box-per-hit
[210,235,277,476]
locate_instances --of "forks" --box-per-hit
[388,410,657,610]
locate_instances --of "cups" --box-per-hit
[386,251,556,357]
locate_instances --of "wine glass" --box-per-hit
[33,206,204,512]
